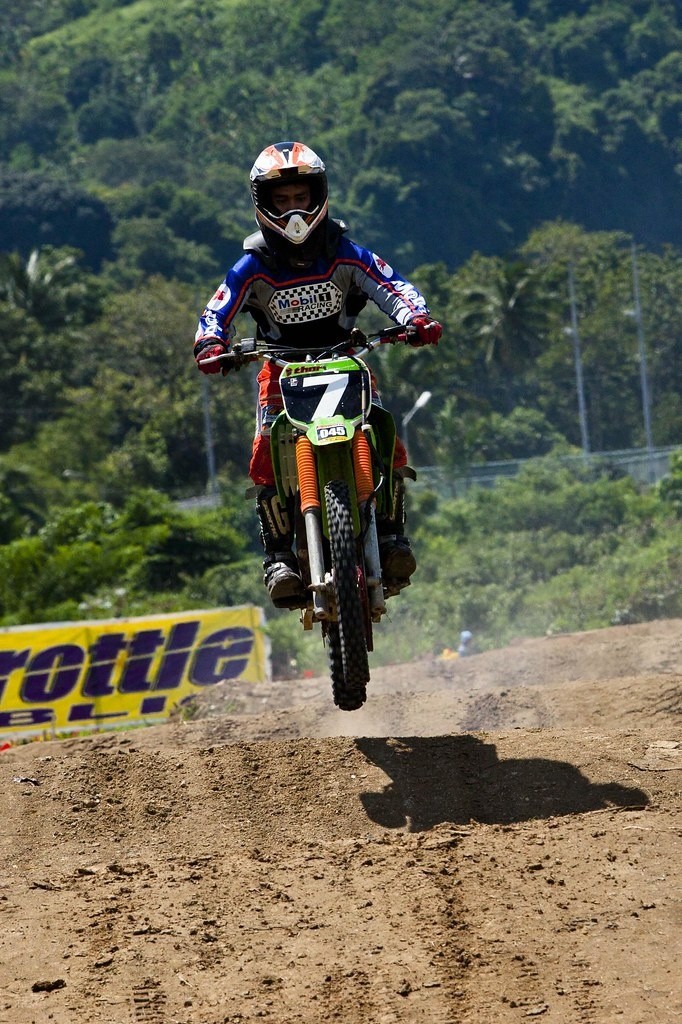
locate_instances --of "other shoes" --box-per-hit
[384,544,416,578]
[263,563,301,598]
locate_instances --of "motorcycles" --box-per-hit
[197,321,429,712]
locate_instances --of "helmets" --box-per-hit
[250,142,329,248]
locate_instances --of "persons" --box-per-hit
[195,142,443,602]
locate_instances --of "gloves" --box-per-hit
[405,317,444,345]
[195,344,233,375]
